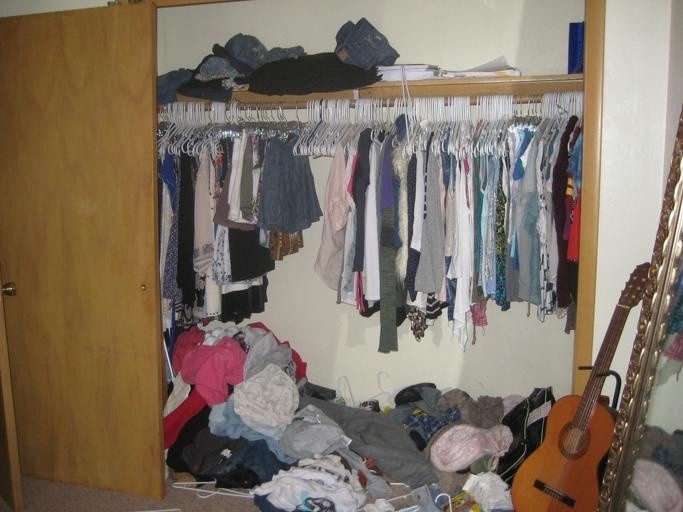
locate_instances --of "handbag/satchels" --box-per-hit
[496,387,555,486]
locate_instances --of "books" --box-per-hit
[374,62,523,83]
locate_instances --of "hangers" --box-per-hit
[356,482,460,512]
[171,477,257,500]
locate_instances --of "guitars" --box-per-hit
[510,263,648,511]
